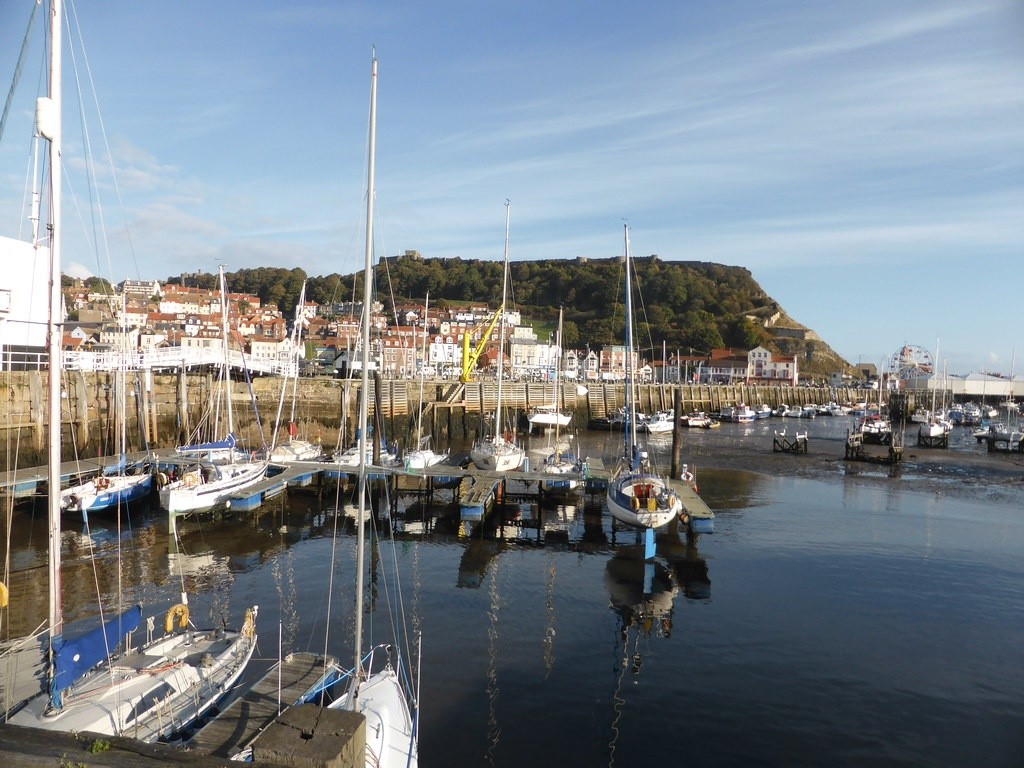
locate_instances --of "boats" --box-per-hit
[1,2,430,768]
[58,43,716,562]
[716,337,1024,470]
[53,494,685,768]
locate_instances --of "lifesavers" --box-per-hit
[157,472,167,484]
[668,495,676,508]
[630,496,639,510]
[680,471,693,482]
[0,581,8,608]
[98,478,109,489]
[164,605,189,633]
[183,473,197,483]
